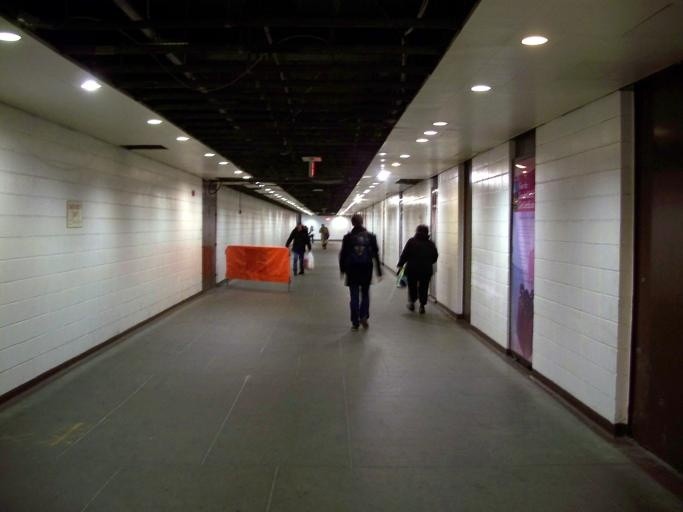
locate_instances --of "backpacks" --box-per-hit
[350,231,371,266]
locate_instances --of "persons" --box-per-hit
[397,224,439,315]
[320,224,329,249]
[339,213,383,331]
[286,222,312,277]
[308,225,315,244]
